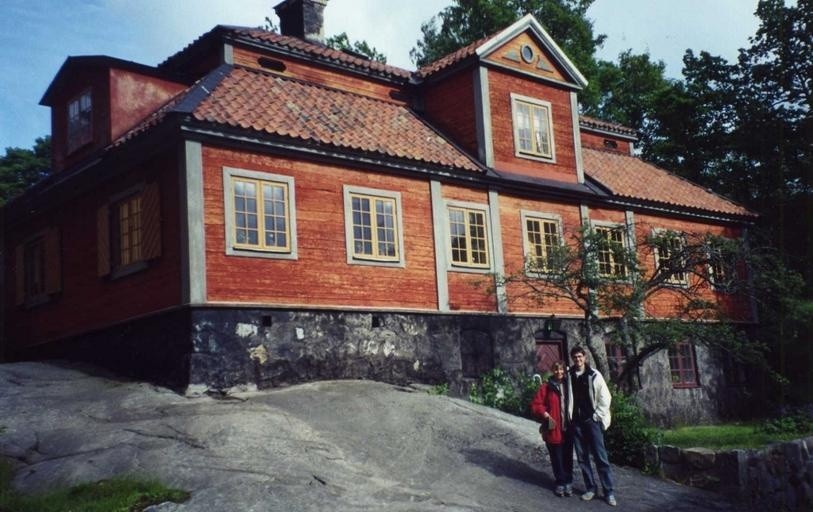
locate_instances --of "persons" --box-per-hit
[560,345,619,506]
[530,361,575,497]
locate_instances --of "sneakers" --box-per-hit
[581,491,596,500]
[605,495,617,506]
[565,483,575,496]
[555,486,565,497]
